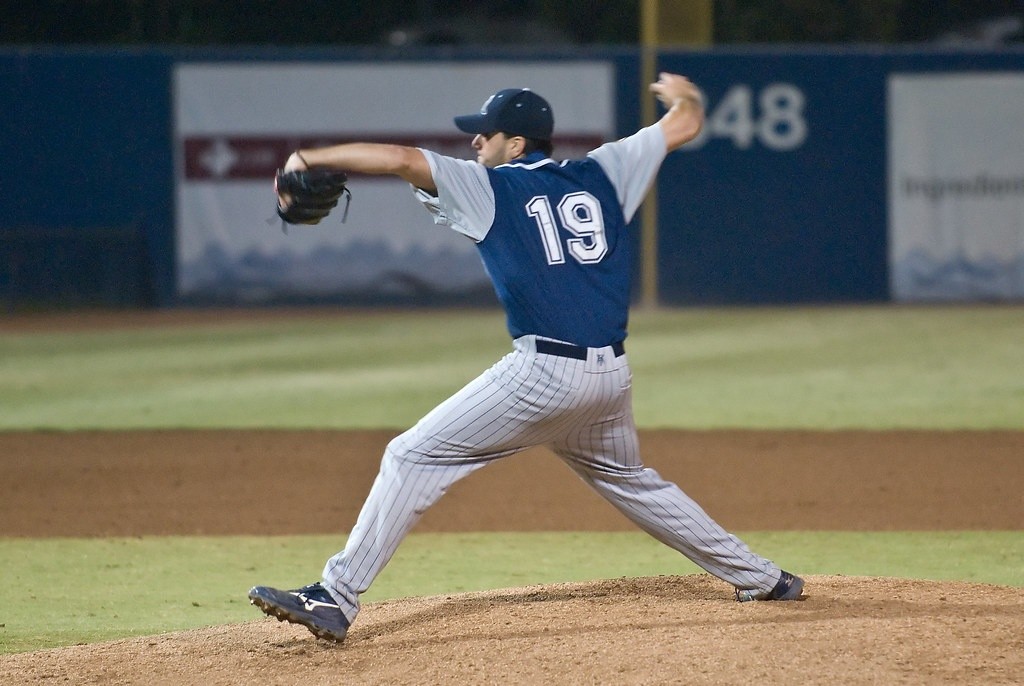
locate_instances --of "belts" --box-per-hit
[536,339,625,362]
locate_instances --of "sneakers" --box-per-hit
[749,570,805,602]
[248,580,350,644]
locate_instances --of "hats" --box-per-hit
[451,86,556,142]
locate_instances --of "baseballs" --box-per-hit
[655,79,665,102]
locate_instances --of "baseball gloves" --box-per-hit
[272,166,352,237]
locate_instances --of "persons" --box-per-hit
[248,72,804,645]
